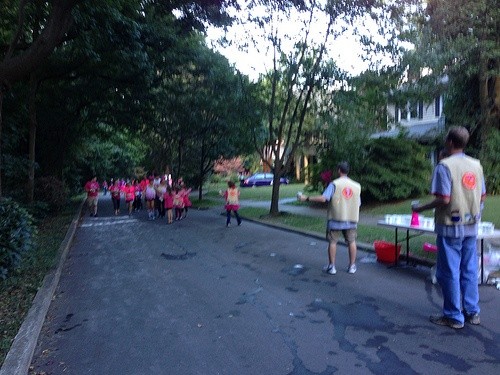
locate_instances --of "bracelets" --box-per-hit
[307,197,309,202]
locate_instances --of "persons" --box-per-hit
[223,181,242,227]
[108,172,192,225]
[238,171,245,186]
[102,179,109,195]
[85,175,100,217]
[411,125,486,329]
[298,161,362,274]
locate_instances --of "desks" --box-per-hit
[377,214,500,286]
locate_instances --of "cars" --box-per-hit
[241,173,289,186]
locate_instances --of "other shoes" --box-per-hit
[430,313,464,329]
[323,264,336,274]
[115,210,188,224]
[465,315,481,325]
[95,214,98,217]
[89,213,93,217]
[348,264,357,274]
